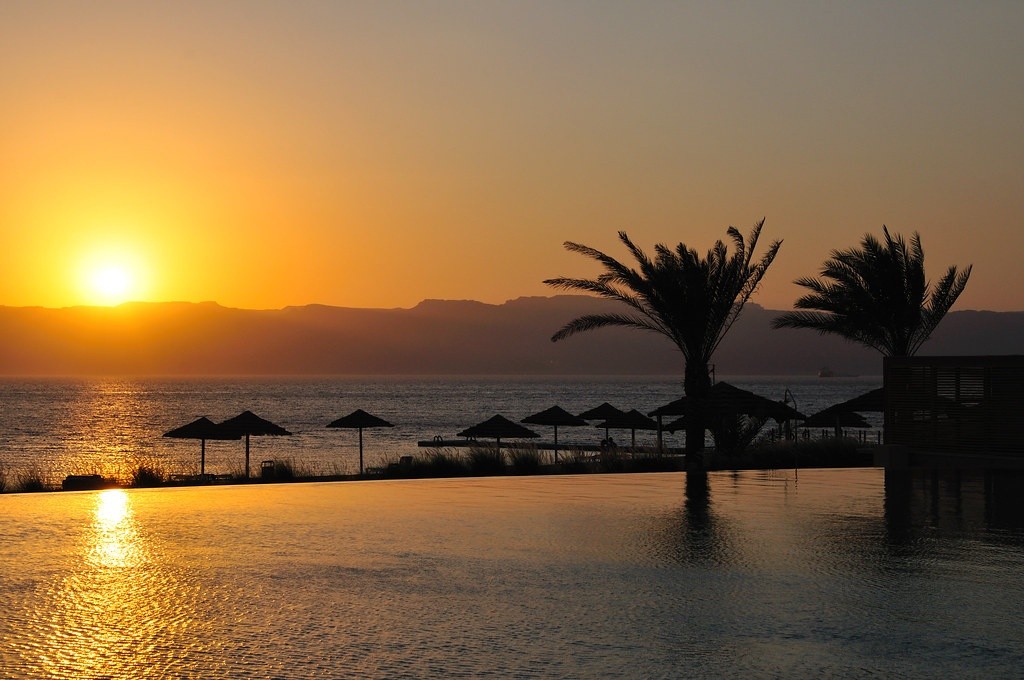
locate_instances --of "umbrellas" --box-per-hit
[520,405,590,462]
[326,409,395,473]
[596,409,663,447]
[800,409,872,440]
[211,411,292,476]
[457,414,542,454]
[162,417,242,473]
[749,401,808,438]
[576,403,630,440]
[660,415,712,430]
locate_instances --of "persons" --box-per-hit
[601,438,617,454]
